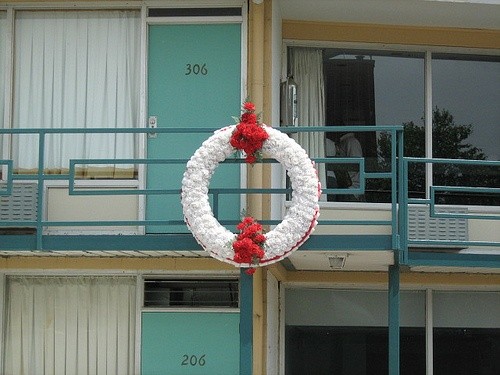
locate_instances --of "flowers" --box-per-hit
[229,94,268,169]
[221,205,266,277]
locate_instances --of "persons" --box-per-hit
[326,133,340,201]
[338,132,365,202]
